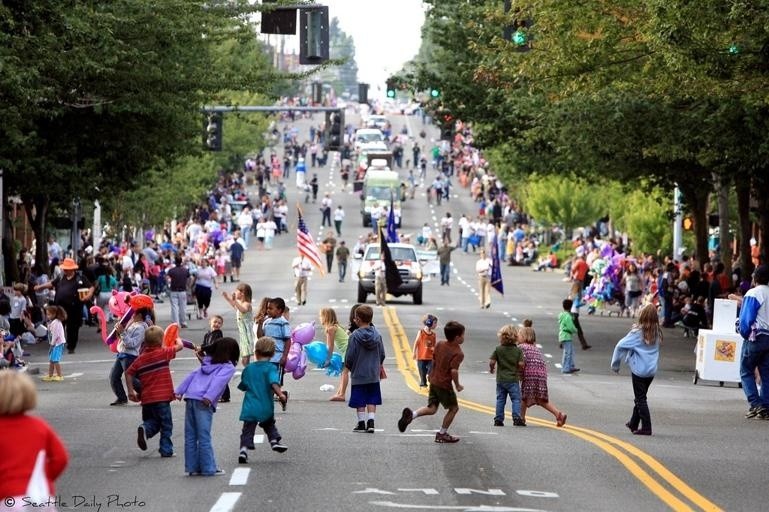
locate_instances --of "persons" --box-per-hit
[1,83,769,511]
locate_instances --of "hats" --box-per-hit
[59,258,79,270]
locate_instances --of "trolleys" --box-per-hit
[592,283,624,318]
[693,329,744,387]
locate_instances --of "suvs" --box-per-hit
[353,245,428,306]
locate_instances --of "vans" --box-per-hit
[358,170,403,229]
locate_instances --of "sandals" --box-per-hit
[557,412,567,427]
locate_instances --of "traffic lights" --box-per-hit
[431,86,440,98]
[708,214,719,227]
[504,2,533,52]
[386,83,395,98]
[680,216,695,231]
[325,110,345,150]
[440,110,455,140]
[203,109,222,151]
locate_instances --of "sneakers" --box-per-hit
[353,419,374,433]
[190,470,226,475]
[138,425,146,450]
[41,375,64,381]
[271,442,288,453]
[239,450,247,463]
[279,391,288,411]
[398,408,413,432]
[626,423,651,435]
[495,419,527,426]
[435,432,459,443]
[745,405,769,420]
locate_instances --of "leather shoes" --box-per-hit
[111,399,128,405]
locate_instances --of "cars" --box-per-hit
[351,114,392,187]
[227,200,249,224]
